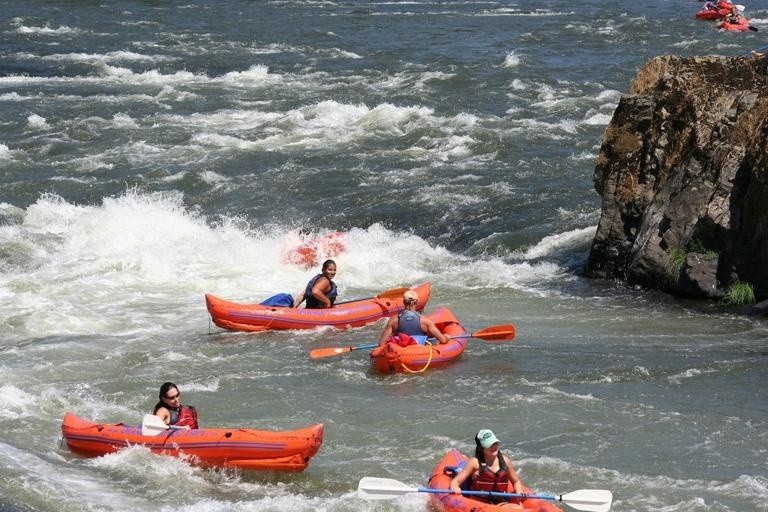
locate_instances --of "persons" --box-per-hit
[702,0,742,25]
[151,380,198,431]
[292,258,338,310]
[377,288,452,350]
[447,427,527,511]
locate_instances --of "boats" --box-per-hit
[288,231,346,269]
[369,303,468,376]
[58,408,325,476]
[694,7,733,21]
[717,16,750,33]
[203,278,433,335]
[426,445,564,512]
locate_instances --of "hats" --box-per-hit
[475,429,501,448]
[403,290,418,302]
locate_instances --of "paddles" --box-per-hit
[310,324,515,358]
[141,413,190,436]
[728,16,758,33]
[357,476,613,511]
[332,287,409,304]
